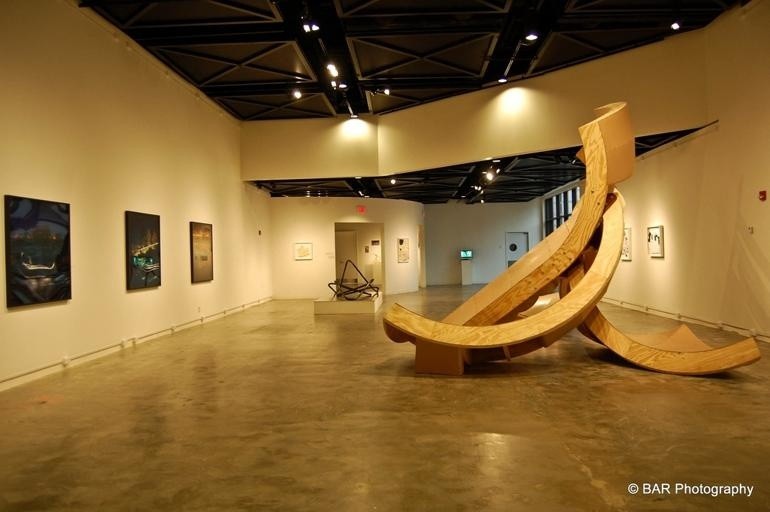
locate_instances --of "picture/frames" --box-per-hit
[620,226,664,261]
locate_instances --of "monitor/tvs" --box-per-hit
[460,249,473,260]
